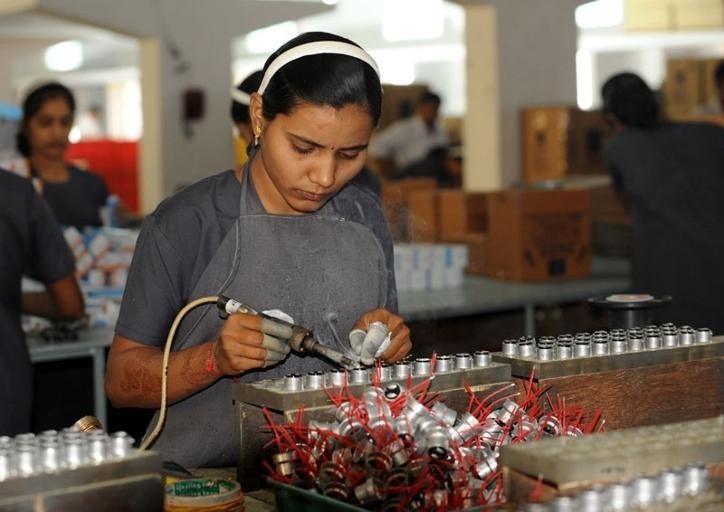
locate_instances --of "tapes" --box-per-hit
[163,477,246,512]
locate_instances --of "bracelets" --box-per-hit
[205,339,222,376]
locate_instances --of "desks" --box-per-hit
[18,275,632,437]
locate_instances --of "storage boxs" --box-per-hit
[372,61,724,286]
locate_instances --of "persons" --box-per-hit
[600,72,724,338]
[395,156,463,186]
[366,93,449,166]
[104,32,412,471]
[229,72,383,200]
[0,168,85,430]
[0,83,113,227]
